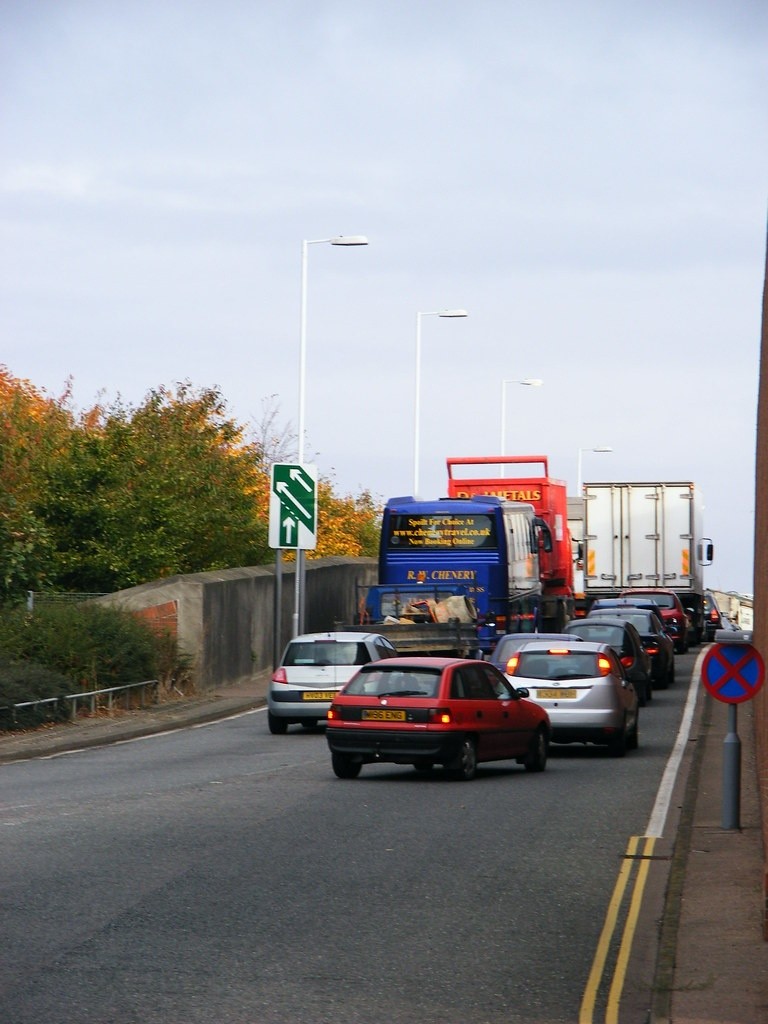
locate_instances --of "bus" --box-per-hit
[377,495,554,641]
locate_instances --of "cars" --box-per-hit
[558,618,658,709]
[585,607,679,691]
[267,631,404,735]
[489,632,584,676]
[323,655,553,783]
[500,640,646,757]
[591,588,731,654]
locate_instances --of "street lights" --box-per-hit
[291,234,371,640]
[579,447,615,497]
[500,377,546,479]
[411,308,469,500]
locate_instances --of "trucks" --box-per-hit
[581,478,713,646]
[444,455,585,633]
[352,578,495,665]
